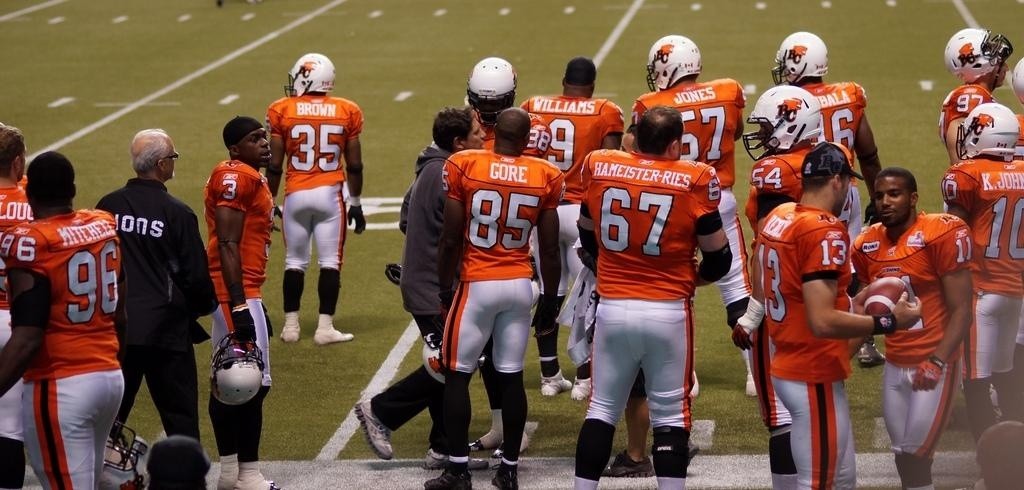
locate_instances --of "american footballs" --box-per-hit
[864,277,910,317]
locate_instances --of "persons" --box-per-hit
[145,436,211,490]
[354,29,1023,490]
[0,149,128,490]
[94,129,221,443]
[203,115,281,490]
[0,124,34,490]
[266,53,366,349]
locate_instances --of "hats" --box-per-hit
[801,142,864,179]
[565,57,595,85]
[223,116,263,148]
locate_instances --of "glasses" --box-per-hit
[154,153,178,166]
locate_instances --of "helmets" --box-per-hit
[100,463,148,490]
[422,333,446,384]
[289,54,336,97]
[944,28,1024,105]
[647,35,701,91]
[956,103,1020,161]
[750,84,821,151]
[209,359,264,405]
[774,31,828,82]
[468,57,516,125]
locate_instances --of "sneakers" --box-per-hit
[423,429,528,490]
[859,342,885,366]
[355,399,392,460]
[280,326,353,346]
[601,450,653,477]
[540,368,592,400]
[218,477,282,490]
[746,379,758,396]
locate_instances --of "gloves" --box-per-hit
[913,357,944,390]
[347,205,365,234]
[233,308,256,341]
[732,324,753,350]
[532,299,557,338]
